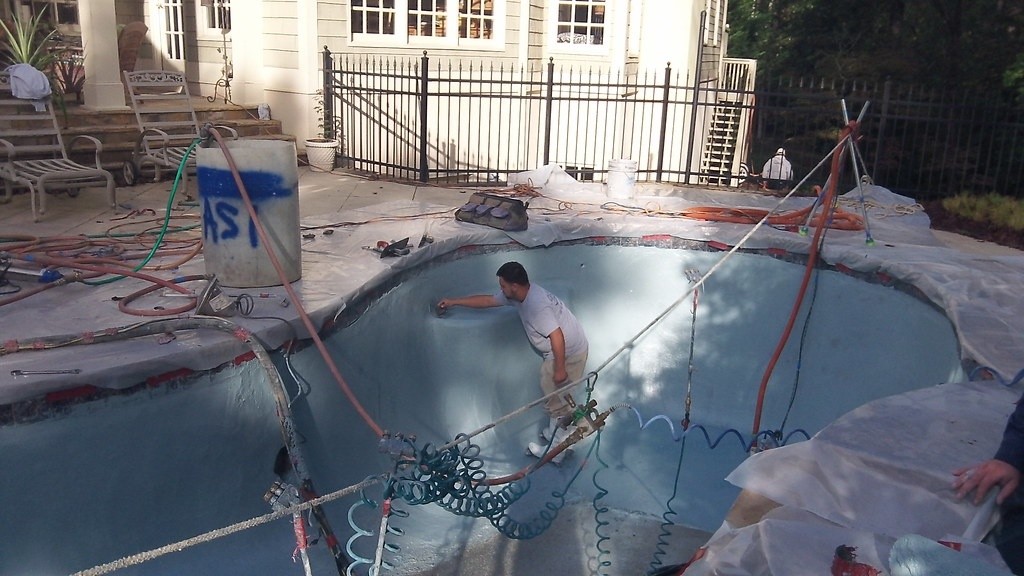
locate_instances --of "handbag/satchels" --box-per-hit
[454,192,530,232]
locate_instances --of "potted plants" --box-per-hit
[0,0,67,114]
[302,90,342,172]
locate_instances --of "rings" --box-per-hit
[965,469,977,479]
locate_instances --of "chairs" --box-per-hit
[0,63,118,223]
[118,21,148,105]
[121,69,238,195]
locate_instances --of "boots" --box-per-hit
[542,417,577,443]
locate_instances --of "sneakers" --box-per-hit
[528,425,577,463]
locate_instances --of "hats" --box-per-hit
[776,148,785,155]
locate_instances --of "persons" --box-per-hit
[438,262,589,464]
[950,392,1024,576]
[763,149,794,191]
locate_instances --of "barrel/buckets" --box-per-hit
[195,140,301,287]
[605,158,638,202]
